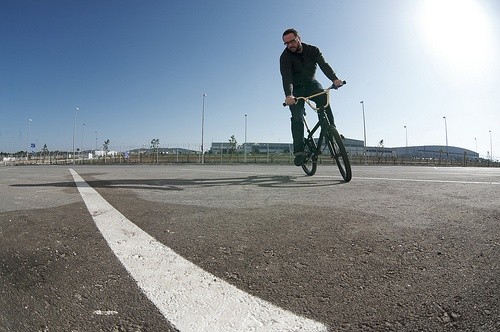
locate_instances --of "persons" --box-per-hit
[279,29,343,167]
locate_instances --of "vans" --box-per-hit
[107,151,118,156]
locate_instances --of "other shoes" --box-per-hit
[294,155,304,166]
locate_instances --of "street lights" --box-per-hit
[28,118,32,160]
[73,107,80,165]
[444,117,448,164]
[360,100,367,164]
[82,124,85,150]
[405,126,408,158]
[475,138,477,152]
[244,115,247,163]
[489,130,493,163]
[202,95,206,164]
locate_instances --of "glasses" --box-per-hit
[284,38,298,46]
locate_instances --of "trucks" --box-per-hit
[94,151,106,157]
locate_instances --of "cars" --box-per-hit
[3,155,16,161]
[17,155,65,161]
[120,154,129,159]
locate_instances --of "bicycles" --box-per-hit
[283,81,352,182]
